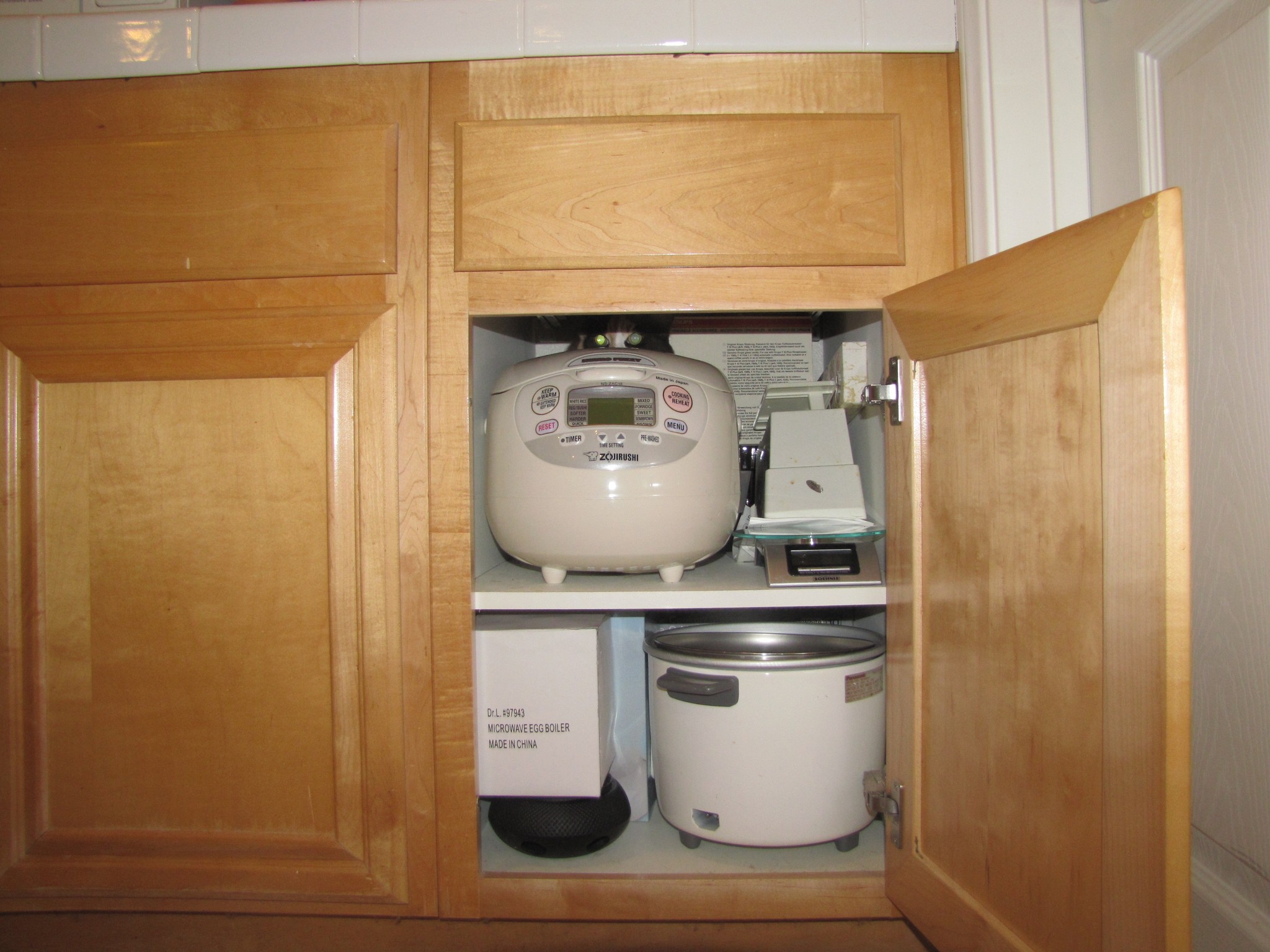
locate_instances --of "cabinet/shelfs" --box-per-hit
[430,52,1197,952]
[0,62,441,918]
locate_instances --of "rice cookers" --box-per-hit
[644,619,888,853]
[484,348,740,586]
